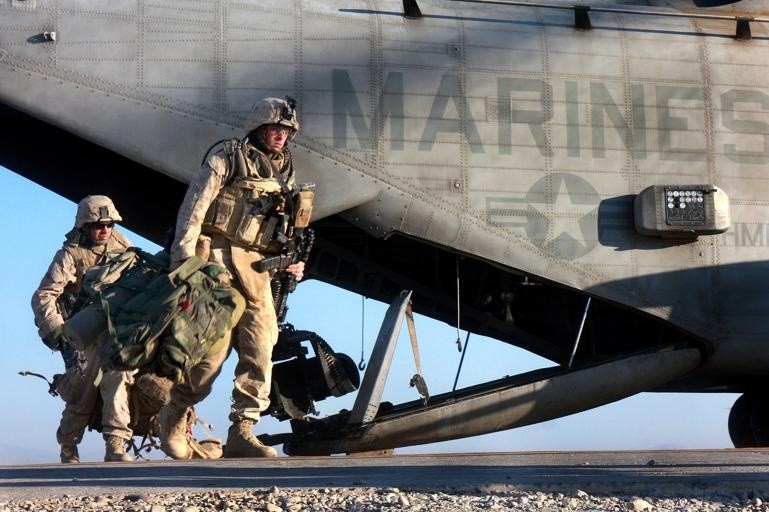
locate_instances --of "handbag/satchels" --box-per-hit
[82,251,137,296]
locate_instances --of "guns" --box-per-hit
[259,228,315,333]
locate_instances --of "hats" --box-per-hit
[245,98,298,138]
[73,195,122,228]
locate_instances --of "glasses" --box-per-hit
[265,124,295,136]
[96,223,115,230]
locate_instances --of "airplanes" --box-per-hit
[2,0,768,457]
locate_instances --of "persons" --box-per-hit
[160,98,306,460]
[30,194,134,464]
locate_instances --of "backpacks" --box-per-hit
[97,255,246,384]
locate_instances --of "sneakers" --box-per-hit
[224,419,277,458]
[159,398,189,460]
[105,435,132,463]
[60,445,80,464]
